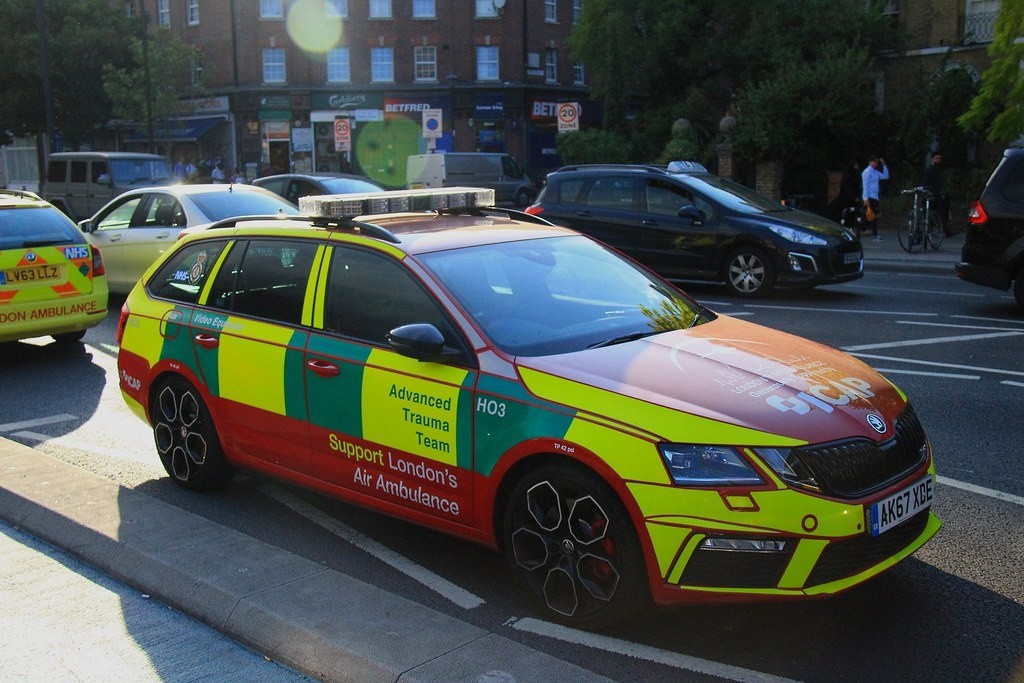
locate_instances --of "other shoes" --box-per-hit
[945,229,961,238]
[872,235,885,242]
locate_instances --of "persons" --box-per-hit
[225,168,247,184]
[98,165,111,181]
[211,163,225,184]
[186,159,196,177]
[858,156,889,242]
[925,154,959,238]
[199,156,223,173]
[174,156,186,178]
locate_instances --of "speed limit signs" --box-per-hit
[334,118,351,144]
[557,103,578,132]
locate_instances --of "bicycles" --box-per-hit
[896,187,943,255]
[840,198,864,232]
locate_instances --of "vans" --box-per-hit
[44,151,178,232]
[405,152,536,207]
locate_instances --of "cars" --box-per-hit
[252,171,393,208]
[955,146,1024,312]
[78,184,302,296]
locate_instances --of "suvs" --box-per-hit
[524,160,864,300]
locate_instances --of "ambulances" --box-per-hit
[0,187,110,344]
[116,195,939,640]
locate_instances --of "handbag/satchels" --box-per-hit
[866,205,875,222]
[841,207,866,231]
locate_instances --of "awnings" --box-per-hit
[124,116,224,144]
